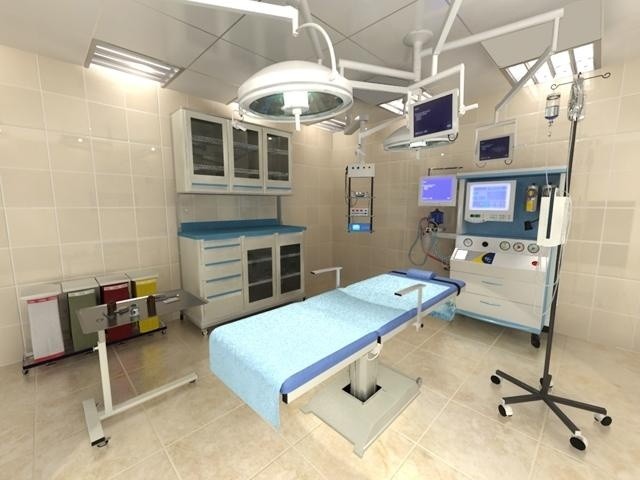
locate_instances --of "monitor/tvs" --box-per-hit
[464,180,517,222]
[476,132,512,164]
[417,174,457,207]
[409,88,459,142]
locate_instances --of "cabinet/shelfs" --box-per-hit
[176,223,307,336]
[171,109,293,197]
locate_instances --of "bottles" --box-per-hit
[544,182,552,197]
[527,182,537,211]
[544,93,560,123]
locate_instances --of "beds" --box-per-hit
[207,268,463,458]
